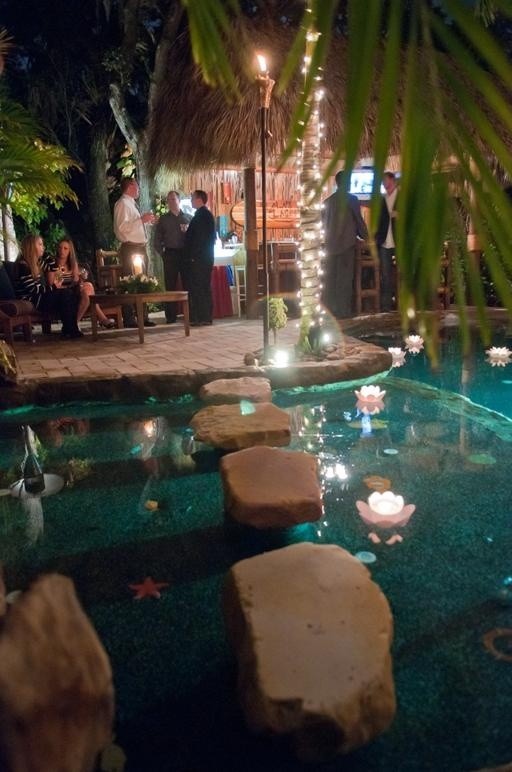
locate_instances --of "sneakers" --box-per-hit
[58,318,83,340]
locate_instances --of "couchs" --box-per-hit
[0,261,123,346]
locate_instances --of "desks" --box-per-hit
[183,265,235,322]
[89,290,190,345]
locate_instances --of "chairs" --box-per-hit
[230,263,269,316]
[94,248,130,316]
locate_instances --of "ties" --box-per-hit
[135,204,147,239]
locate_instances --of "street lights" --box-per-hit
[244,53,286,367]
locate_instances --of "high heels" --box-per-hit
[99,320,118,330]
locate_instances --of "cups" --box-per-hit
[179,223,187,232]
[229,235,237,248]
[272,236,296,242]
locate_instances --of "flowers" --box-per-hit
[117,274,164,294]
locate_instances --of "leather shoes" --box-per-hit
[166,318,177,324]
[122,316,138,328]
[189,316,213,327]
[143,320,157,327]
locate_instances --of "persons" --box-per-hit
[375,171,401,314]
[320,170,371,319]
[178,189,217,329]
[153,189,194,326]
[111,177,161,330]
[47,239,117,336]
[11,234,86,340]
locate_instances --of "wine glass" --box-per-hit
[146,209,160,226]
[77,266,87,285]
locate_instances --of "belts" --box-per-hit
[124,242,147,247]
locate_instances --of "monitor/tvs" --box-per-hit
[348,168,403,201]
